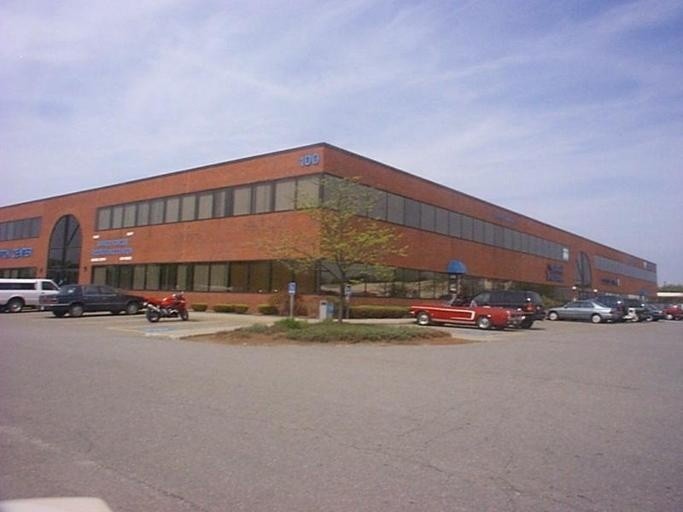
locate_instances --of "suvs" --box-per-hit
[467,289,546,329]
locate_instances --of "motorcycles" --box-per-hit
[142,284,188,323]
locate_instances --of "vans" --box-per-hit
[0,278,62,313]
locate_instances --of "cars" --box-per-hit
[408,294,525,331]
[592,293,682,323]
[38,284,148,317]
[547,299,616,324]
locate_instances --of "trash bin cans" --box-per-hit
[319,299,334,322]
